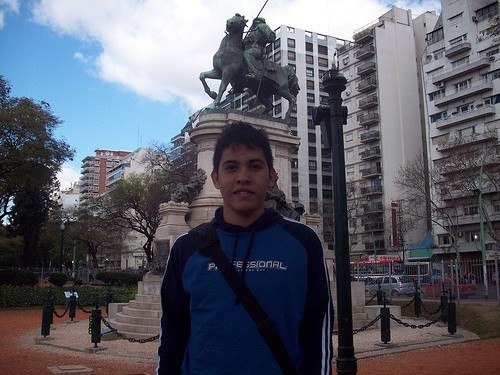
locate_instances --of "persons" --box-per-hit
[241,18,277,80]
[155,122,334,375]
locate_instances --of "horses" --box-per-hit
[199,13,297,123]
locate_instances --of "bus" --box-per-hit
[350,259,405,282]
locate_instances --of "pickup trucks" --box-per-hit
[416,278,477,300]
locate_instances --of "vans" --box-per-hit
[364,274,418,297]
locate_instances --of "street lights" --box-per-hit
[59,222,65,272]
[352,216,377,260]
